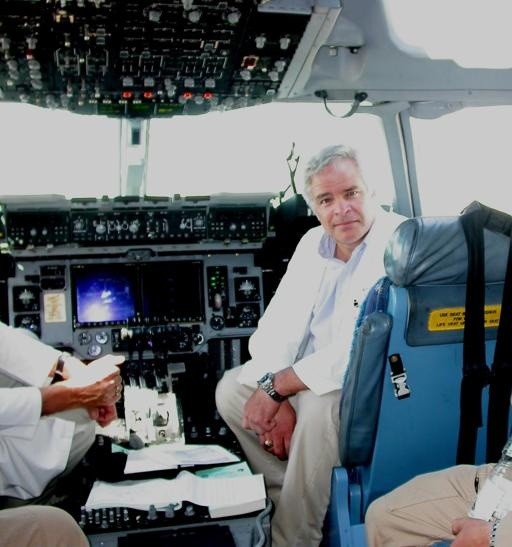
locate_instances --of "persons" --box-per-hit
[214,139,407,546]
[364,424,512,546]
[0,316,127,502]
[1,501,96,546]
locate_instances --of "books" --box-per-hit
[88,442,268,516]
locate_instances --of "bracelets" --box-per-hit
[51,350,73,386]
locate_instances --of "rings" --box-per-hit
[264,439,275,446]
[115,385,120,396]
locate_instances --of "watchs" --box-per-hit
[258,373,289,402]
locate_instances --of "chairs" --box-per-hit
[329,215,512,546]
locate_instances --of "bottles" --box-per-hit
[468,439,512,528]
